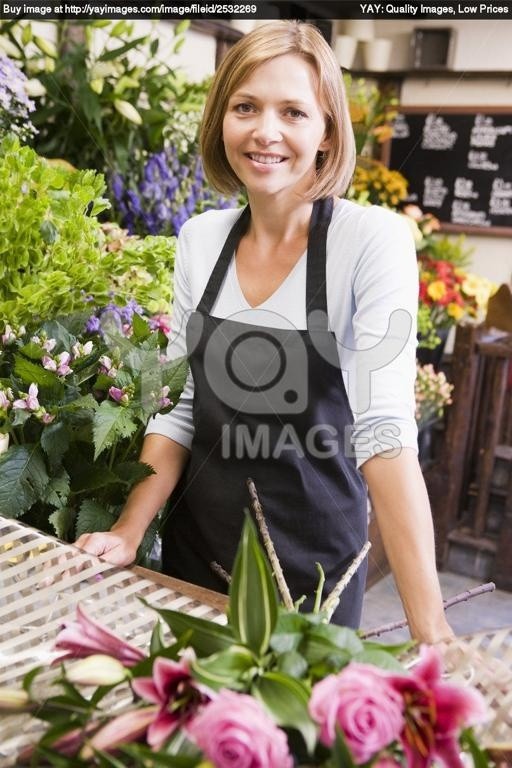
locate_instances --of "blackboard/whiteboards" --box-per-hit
[383,105,511,239]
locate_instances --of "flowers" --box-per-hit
[2,479,512,766]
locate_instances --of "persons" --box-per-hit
[69,20,454,649]
